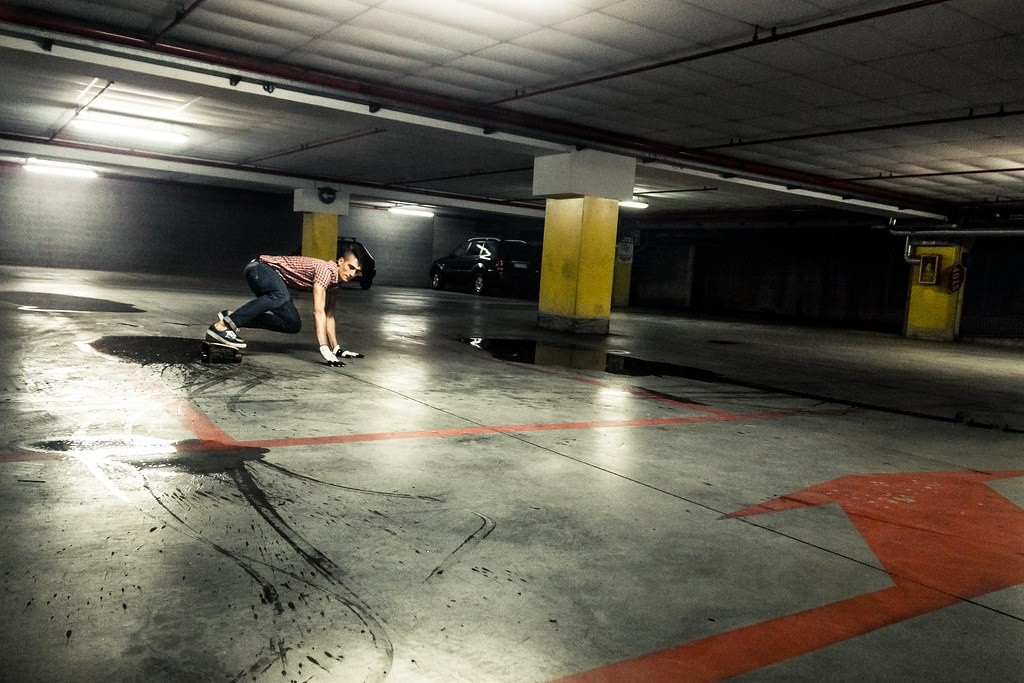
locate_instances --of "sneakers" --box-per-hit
[217,309,240,333]
[206,322,247,348]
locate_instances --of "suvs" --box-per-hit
[289,236,375,289]
[429,237,538,297]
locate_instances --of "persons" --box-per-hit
[206,245,366,368]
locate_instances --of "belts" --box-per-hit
[257,258,265,264]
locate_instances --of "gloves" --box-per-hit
[319,345,346,366]
[332,345,365,358]
